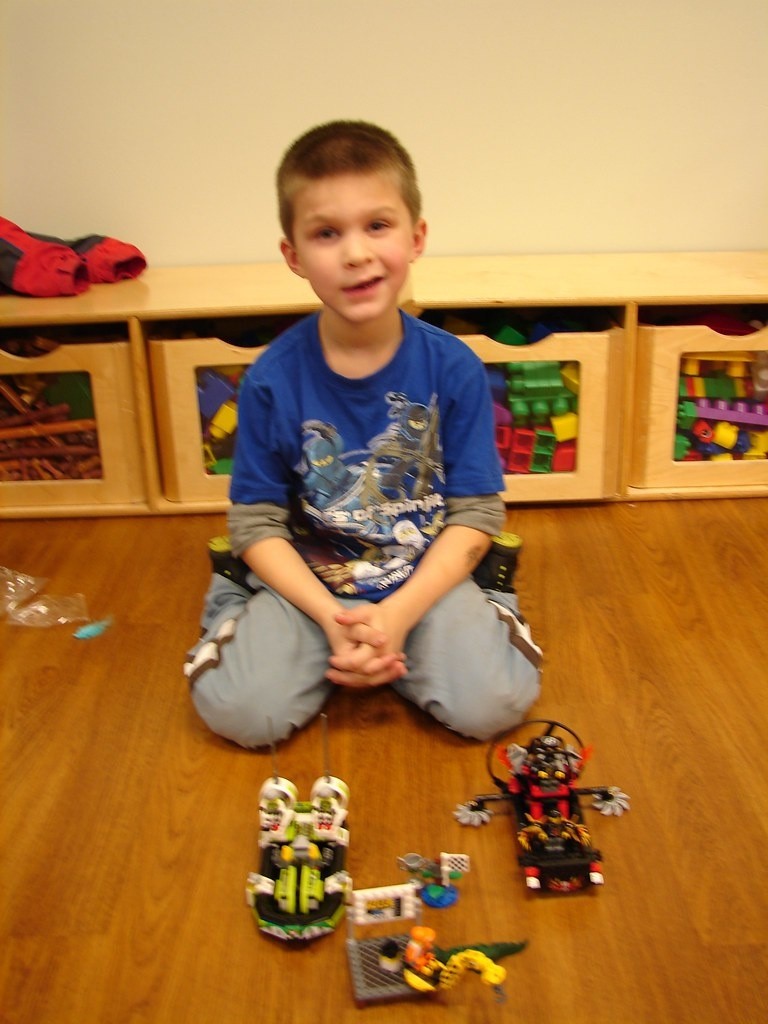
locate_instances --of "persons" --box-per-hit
[402,927,438,972]
[183,119,550,752]
[531,733,567,791]
[539,810,572,851]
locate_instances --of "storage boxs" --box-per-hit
[0,340,145,507]
[446,320,625,505]
[632,323,768,493]
[144,337,273,504]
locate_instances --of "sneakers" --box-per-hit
[207,535,249,592]
[472,531,523,595]
